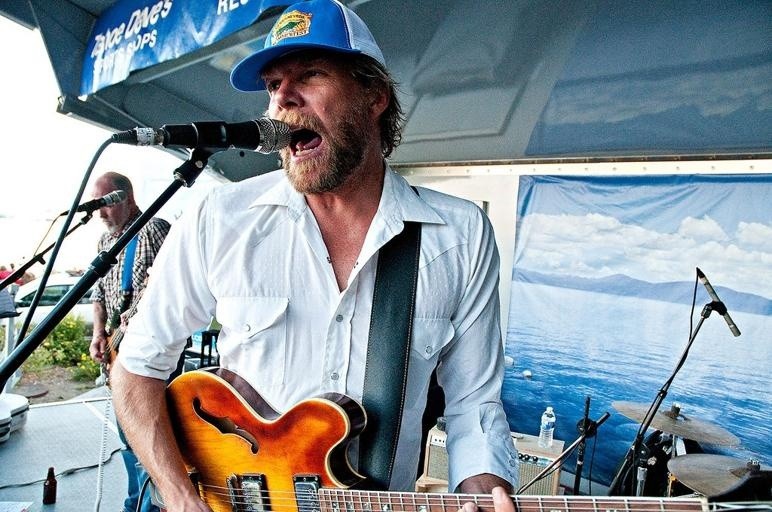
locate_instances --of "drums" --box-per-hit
[612,430,704,497]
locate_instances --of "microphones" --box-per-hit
[61,188,128,215]
[113,116,294,156]
[697,268,743,338]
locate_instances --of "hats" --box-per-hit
[229,0,387,92]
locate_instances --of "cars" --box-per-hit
[0,271,94,325]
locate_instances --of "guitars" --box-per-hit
[96,269,150,372]
[153,366,770,510]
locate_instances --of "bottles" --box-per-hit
[42,467,57,505]
[539,407,558,449]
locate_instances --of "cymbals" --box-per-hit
[667,454,772,502]
[612,402,740,446]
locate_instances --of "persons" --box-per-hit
[107,2,520,512]
[0,263,25,286]
[90,172,171,512]
